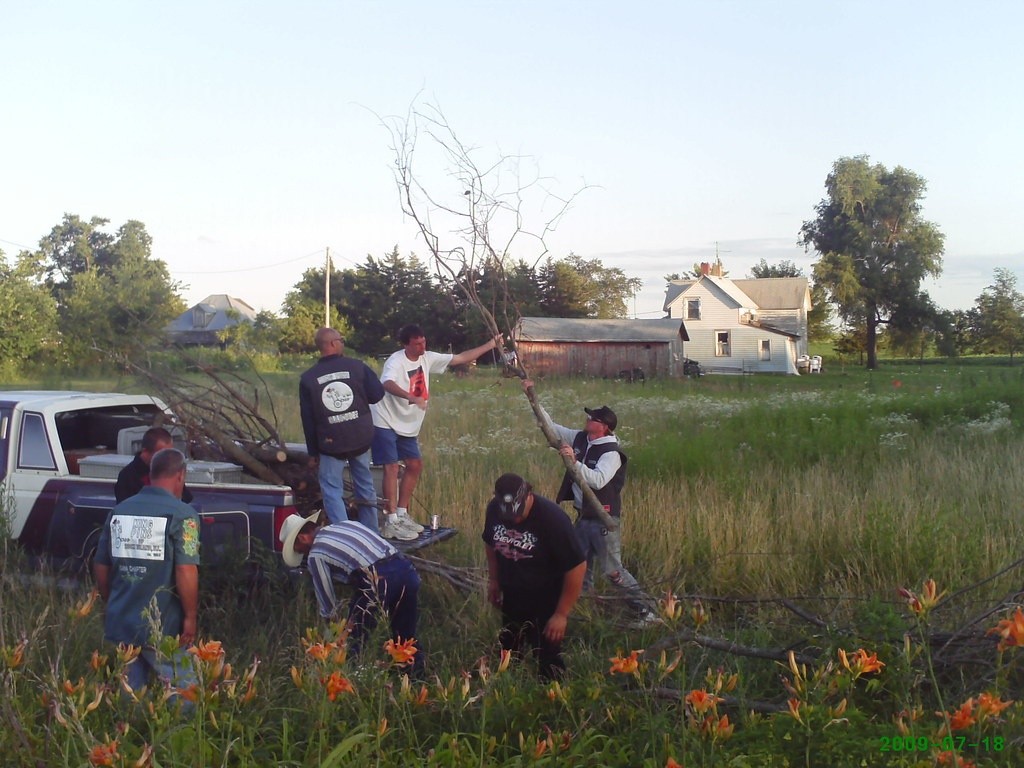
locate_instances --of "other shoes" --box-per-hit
[628,608,660,631]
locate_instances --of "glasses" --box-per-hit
[588,415,606,423]
[333,338,346,343]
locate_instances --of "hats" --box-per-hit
[584,406,617,431]
[278,508,321,567]
[494,473,528,520]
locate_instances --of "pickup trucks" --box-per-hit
[0,391,457,605]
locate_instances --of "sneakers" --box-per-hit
[381,520,419,541]
[395,513,425,533]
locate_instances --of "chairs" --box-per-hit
[797,353,823,374]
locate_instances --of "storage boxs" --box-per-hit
[64,448,116,475]
[117,424,189,461]
[76,453,135,478]
[185,457,243,484]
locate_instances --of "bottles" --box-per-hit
[429,513,440,530]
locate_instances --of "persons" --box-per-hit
[94,426,203,713]
[370,325,504,541]
[278,509,425,685]
[521,378,659,628]
[298,327,385,536]
[481,472,587,680]
